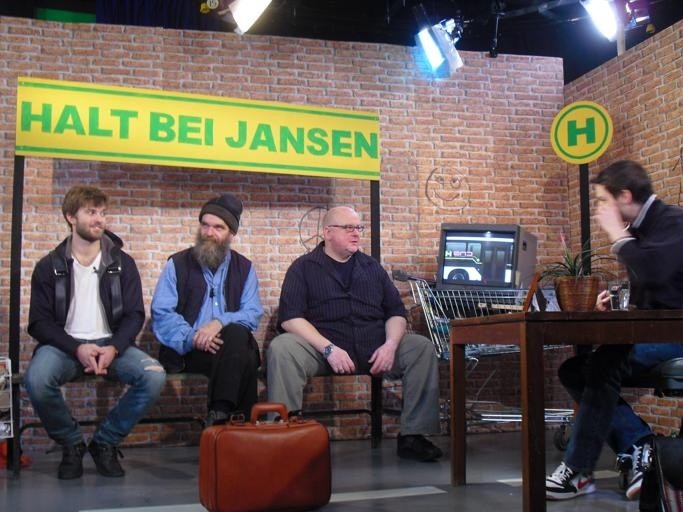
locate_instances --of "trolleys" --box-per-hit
[390,268,574,451]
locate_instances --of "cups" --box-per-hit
[607,279,631,312]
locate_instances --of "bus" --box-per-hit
[443,236,514,284]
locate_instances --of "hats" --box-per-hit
[197,194,243,234]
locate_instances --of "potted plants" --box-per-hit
[526,237,617,311]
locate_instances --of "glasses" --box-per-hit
[326,224,365,233]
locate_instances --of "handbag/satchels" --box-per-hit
[197,403,332,511]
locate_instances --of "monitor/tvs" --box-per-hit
[436,221,537,308]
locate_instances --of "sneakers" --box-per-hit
[87,439,127,478]
[396,433,442,462]
[545,461,597,500]
[56,442,87,479]
[614,434,655,501]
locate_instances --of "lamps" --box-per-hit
[226,0,302,37]
[410,14,483,80]
[580,0,651,56]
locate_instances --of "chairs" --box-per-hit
[552,350,683,491]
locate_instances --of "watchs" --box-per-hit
[323,343,335,359]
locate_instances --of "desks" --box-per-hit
[448,310,683,512]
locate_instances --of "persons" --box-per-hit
[25,184,166,480]
[150,196,261,424]
[545,159,683,501]
[267,207,444,461]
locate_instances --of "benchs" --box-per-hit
[10,362,395,479]
